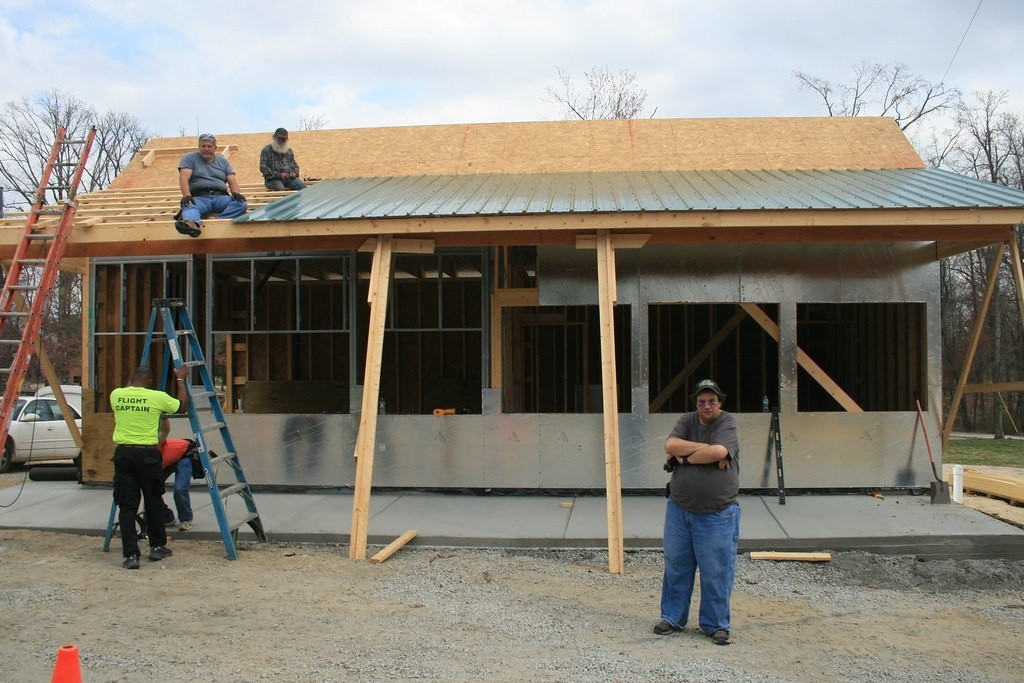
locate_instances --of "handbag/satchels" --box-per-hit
[189,440,205,479]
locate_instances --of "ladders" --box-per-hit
[103,297,268,560]
[0,124,96,456]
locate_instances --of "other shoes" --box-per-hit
[175,221,201,238]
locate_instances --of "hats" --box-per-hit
[276,128,288,139]
[690,379,722,398]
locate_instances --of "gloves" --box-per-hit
[231,192,247,203]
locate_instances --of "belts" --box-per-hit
[117,444,157,449]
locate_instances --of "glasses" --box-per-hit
[199,134,212,138]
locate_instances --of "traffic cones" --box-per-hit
[51,645,82,683]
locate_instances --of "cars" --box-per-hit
[0,385,83,473]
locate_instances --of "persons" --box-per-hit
[174,134,249,238]
[157,438,194,530]
[654,379,741,643]
[110,364,188,570]
[260,128,308,192]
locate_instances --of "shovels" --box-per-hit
[913,389,951,504]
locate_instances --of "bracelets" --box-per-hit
[177,378,185,381]
[682,455,692,465]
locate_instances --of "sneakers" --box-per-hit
[654,620,674,634]
[179,519,194,530]
[164,518,176,527]
[711,629,731,644]
[122,554,139,570]
[149,546,173,561]
